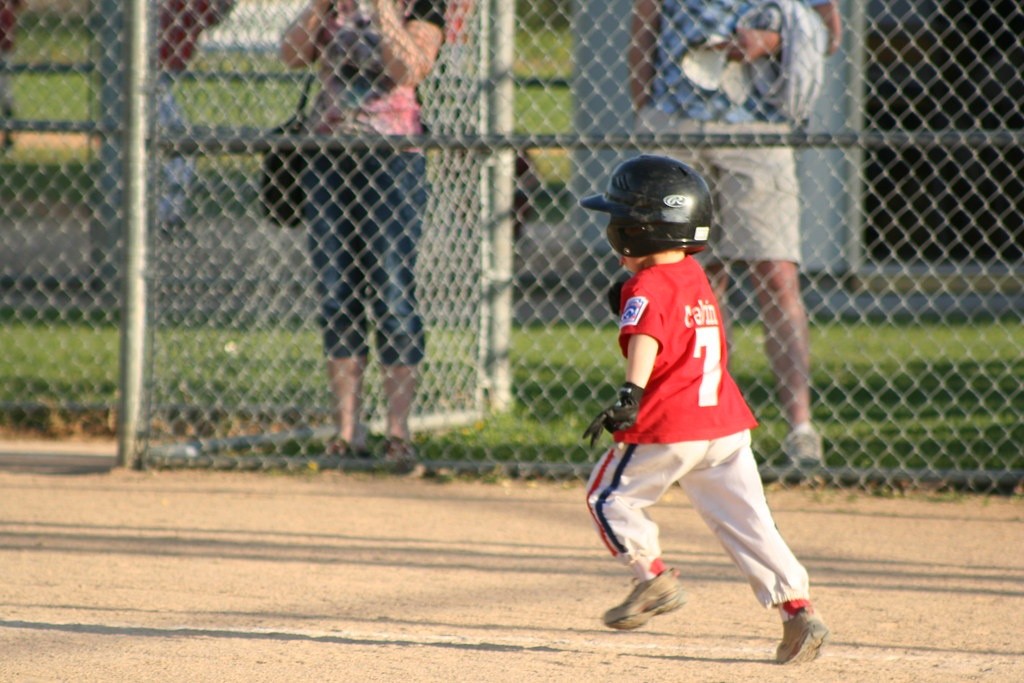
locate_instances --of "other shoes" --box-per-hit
[784,426,825,468]
[378,435,414,462]
[319,428,372,457]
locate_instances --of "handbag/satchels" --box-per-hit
[259,112,315,230]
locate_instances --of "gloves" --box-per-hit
[581,382,645,449]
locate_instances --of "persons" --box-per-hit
[626,0,844,466]
[278,1,444,473]
[580,151,829,666]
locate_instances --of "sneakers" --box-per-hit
[775,608,829,664]
[603,567,687,630]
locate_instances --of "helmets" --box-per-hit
[579,154,714,258]
